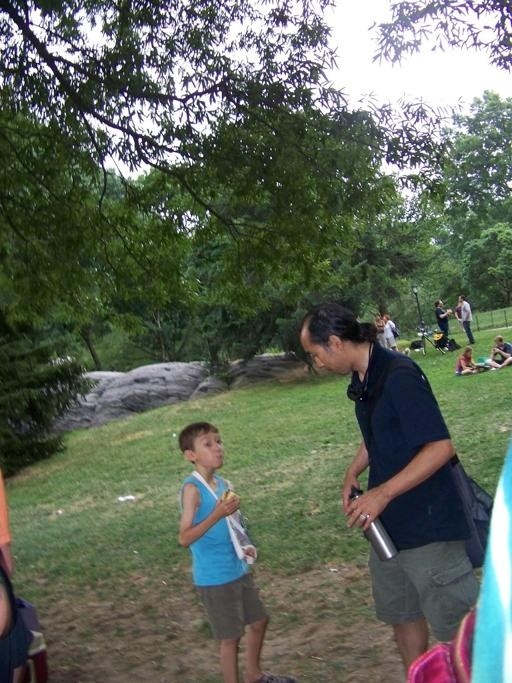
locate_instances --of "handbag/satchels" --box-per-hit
[448,338,462,352]
[221,506,257,564]
[450,460,492,568]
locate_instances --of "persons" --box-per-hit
[459,294,476,345]
[1,475,15,576]
[301,303,495,683]
[470,443,512,683]
[455,300,466,332]
[435,299,453,350]
[455,347,485,375]
[489,336,511,369]
[382,313,398,352]
[178,419,297,682]
[374,312,387,349]
[0,574,49,683]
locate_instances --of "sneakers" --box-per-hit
[255,669,298,683]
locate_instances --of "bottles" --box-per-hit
[347,486,398,562]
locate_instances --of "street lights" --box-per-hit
[410,284,428,357]
